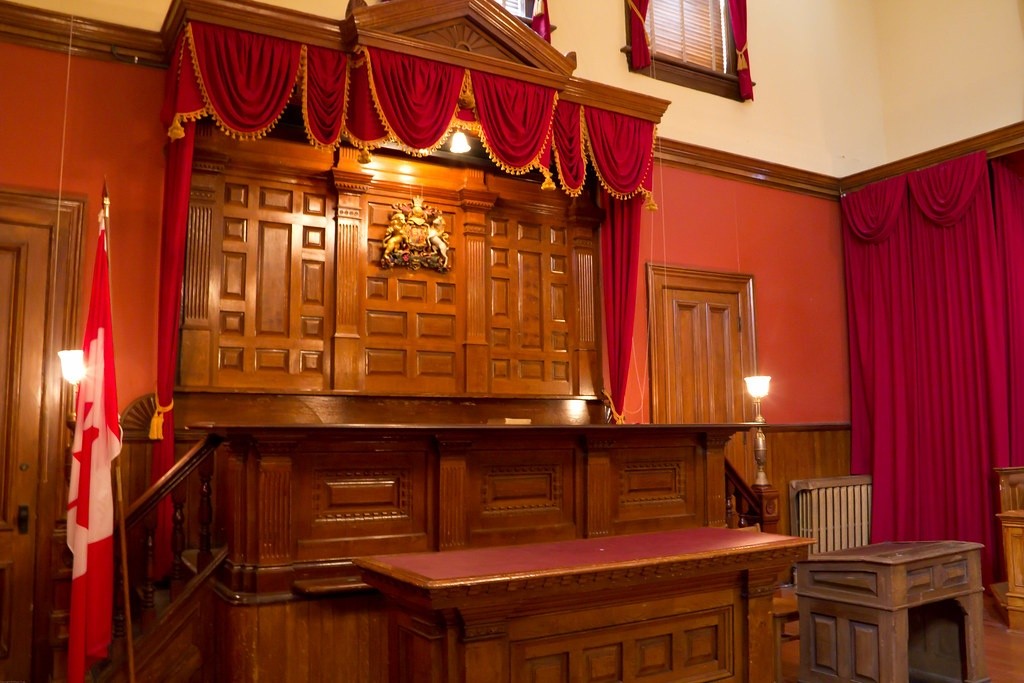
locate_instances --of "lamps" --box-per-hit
[742,374,772,491]
[58,350,88,487]
[449,127,472,154]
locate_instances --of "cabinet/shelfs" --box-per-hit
[353,525,819,683]
[992,465,1024,633]
[794,539,991,683]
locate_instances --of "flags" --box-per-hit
[64,216,124,683]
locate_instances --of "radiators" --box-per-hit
[788,474,872,552]
